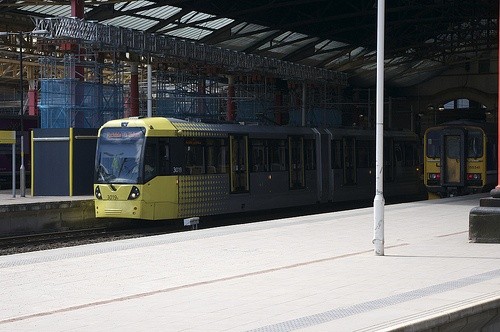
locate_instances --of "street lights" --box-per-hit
[0,30,49,197]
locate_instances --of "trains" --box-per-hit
[423,119,498,199]
[93,116,424,221]
